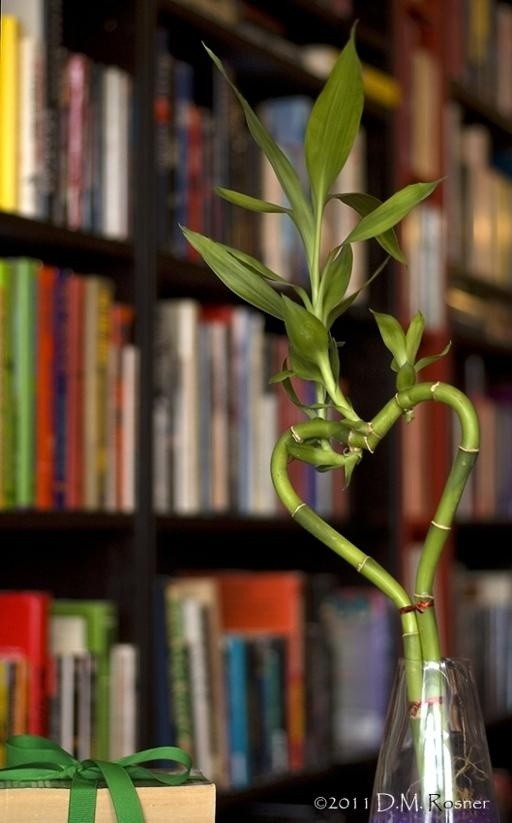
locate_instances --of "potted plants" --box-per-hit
[177,33,497,823]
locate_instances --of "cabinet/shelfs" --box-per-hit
[1,0,402,822]
[398,0,512,731]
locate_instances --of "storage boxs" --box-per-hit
[1,778,217,821]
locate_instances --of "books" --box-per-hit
[164,571,393,792]
[452,567,512,727]
[456,355,511,522]
[446,100,511,298]
[0,13,131,245]
[462,0,512,117]
[150,52,373,310]
[149,299,352,521]
[0,589,139,765]
[0,256,139,515]
